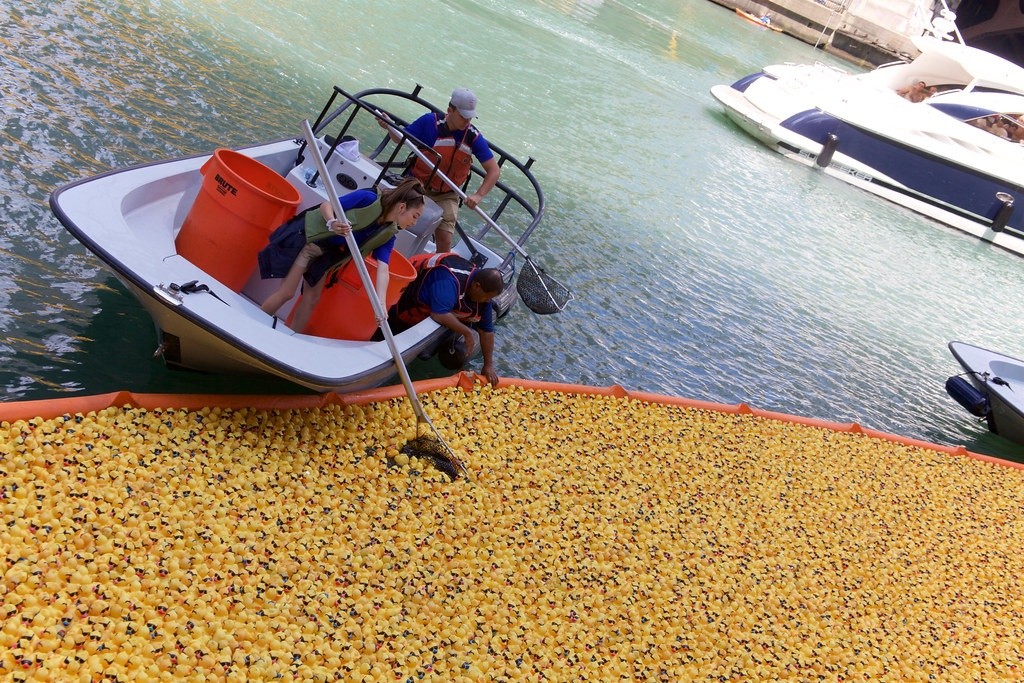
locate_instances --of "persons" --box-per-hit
[761,13,771,24]
[375,88,502,255]
[392,252,504,388]
[258,176,426,336]
[967,114,1024,148]
[896,81,938,103]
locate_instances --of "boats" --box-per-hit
[711,37,1024,263]
[948,340,1024,448]
[50,82,551,395]
[711,0,968,76]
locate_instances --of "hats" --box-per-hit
[450,87,478,119]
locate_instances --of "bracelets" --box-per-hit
[326,218,338,232]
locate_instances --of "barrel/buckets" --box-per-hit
[283,242,418,341]
[173,148,302,295]
